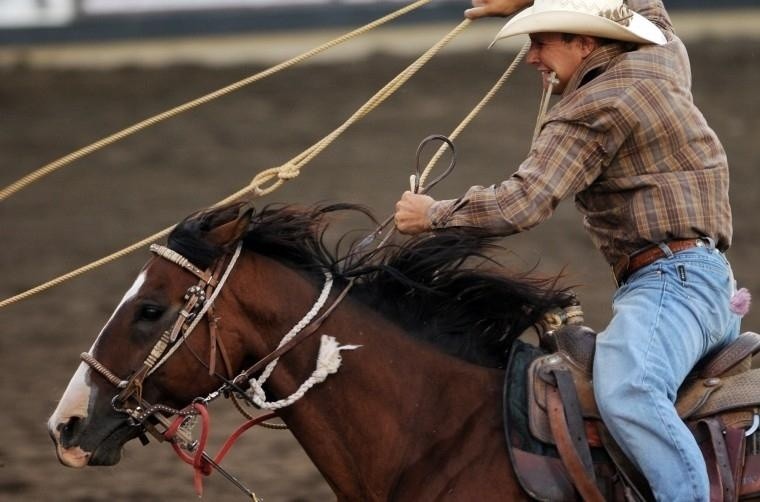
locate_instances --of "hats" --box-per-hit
[486,0,670,50]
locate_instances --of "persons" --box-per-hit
[391,1,741,502]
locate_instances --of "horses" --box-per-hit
[43,196,760,502]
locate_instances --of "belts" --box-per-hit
[609,237,708,288]
